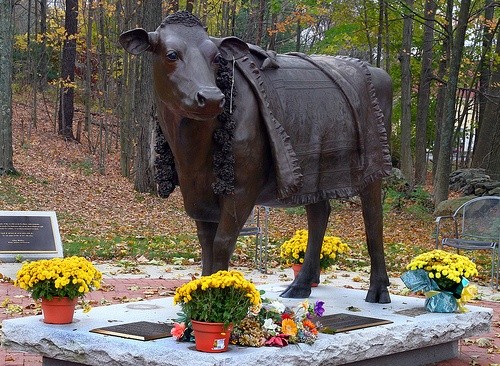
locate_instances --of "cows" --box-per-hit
[115,8,395,306]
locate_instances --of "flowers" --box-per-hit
[404,248,478,292]
[279,229,352,271]
[170,290,326,348]
[172,268,262,328]
[14,256,103,314]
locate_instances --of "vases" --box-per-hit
[291,264,323,288]
[191,319,232,352]
[41,296,77,325]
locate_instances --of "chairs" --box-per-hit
[435,195,500,291]
